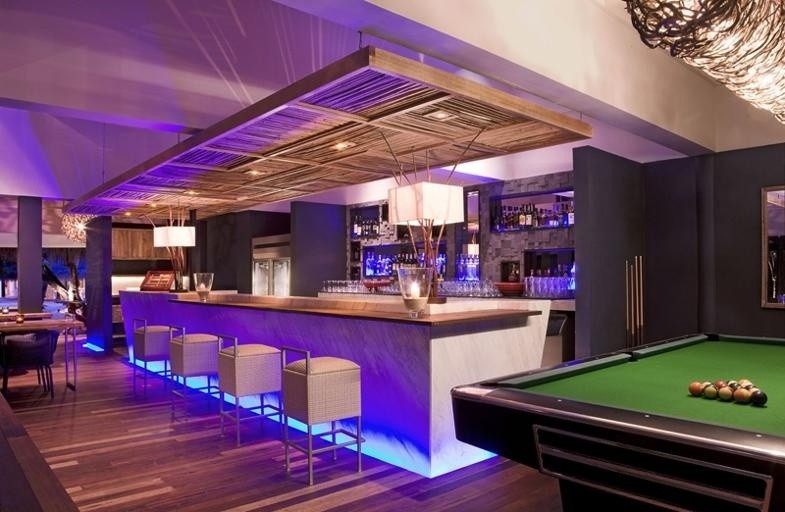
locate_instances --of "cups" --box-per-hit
[193,272,215,301]
[2,305,9,314]
[436,280,500,297]
[524,276,575,298]
[16,315,24,324]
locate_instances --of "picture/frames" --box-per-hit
[759,183,785,310]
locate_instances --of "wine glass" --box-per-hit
[366,278,400,294]
[320,279,367,293]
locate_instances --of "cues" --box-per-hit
[626,255,644,348]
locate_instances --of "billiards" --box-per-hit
[689,378,767,405]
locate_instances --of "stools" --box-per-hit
[217,334,288,446]
[168,323,223,425]
[280,346,365,486]
[131,315,177,397]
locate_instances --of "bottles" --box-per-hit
[351,246,359,261]
[456,253,480,280]
[508,263,518,283]
[352,215,379,238]
[352,267,360,279]
[366,251,446,277]
[501,201,574,227]
[528,263,572,276]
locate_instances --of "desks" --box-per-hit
[0,311,87,399]
[450,331,785,511]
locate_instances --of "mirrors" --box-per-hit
[454,188,481,281]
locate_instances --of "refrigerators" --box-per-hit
[251,232,292,296]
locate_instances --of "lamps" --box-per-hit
[389,183,466,305]
[150,225,196,293]
[619,0,785,128]
[59,200,98,242]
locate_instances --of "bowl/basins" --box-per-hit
[494,282,525,296]
